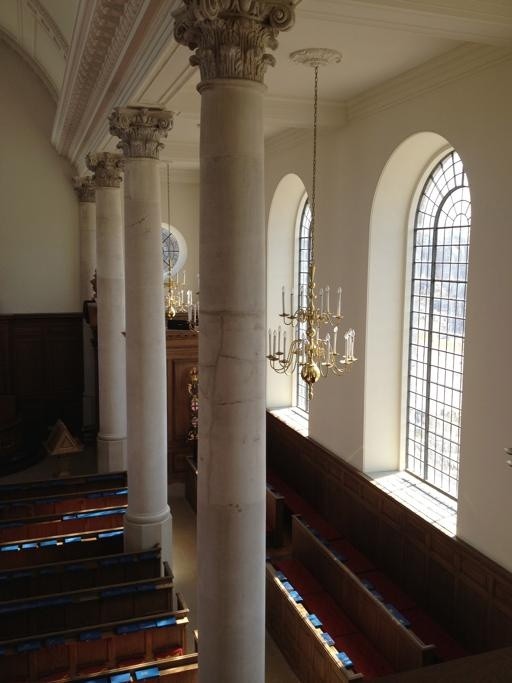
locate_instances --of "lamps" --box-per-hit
[267,49,359,401]
[164,159,198,335]
